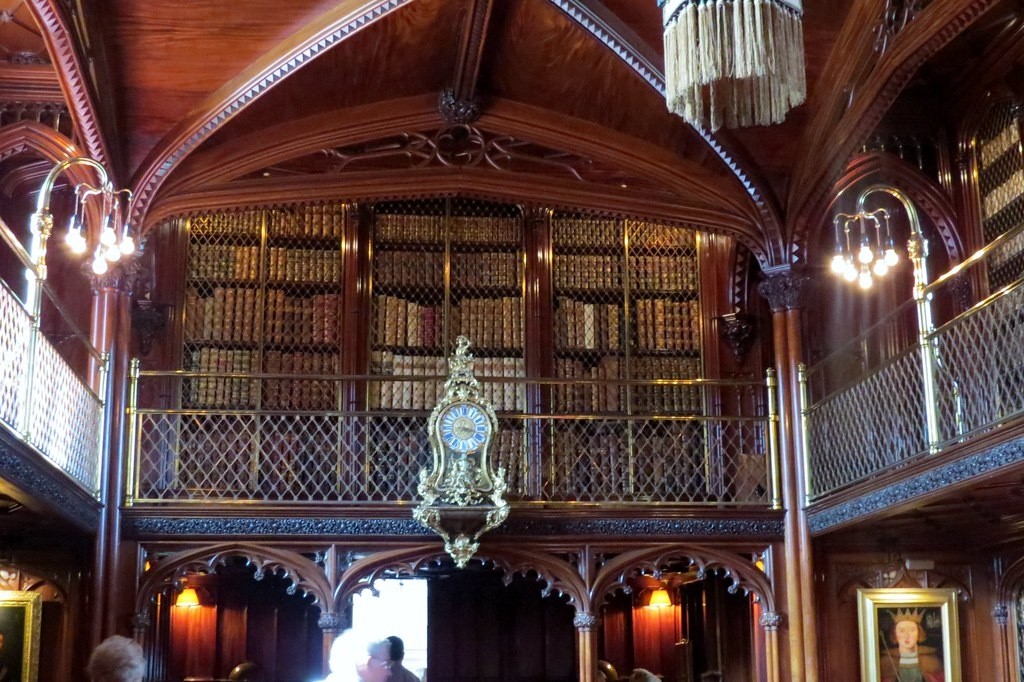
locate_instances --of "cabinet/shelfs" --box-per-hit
[166,199,724,503]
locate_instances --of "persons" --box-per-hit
[356,635,421,682]
[88,636,145,682]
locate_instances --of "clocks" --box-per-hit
[410,334,512,570]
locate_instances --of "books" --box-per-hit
[176,205,707,504]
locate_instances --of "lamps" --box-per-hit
[649,586,673,609]
[175,586,199,606]
[25,155,138,330]
[833,184,942,458]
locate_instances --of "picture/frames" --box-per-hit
[0,590,41,682]
[856,587,962,682]
